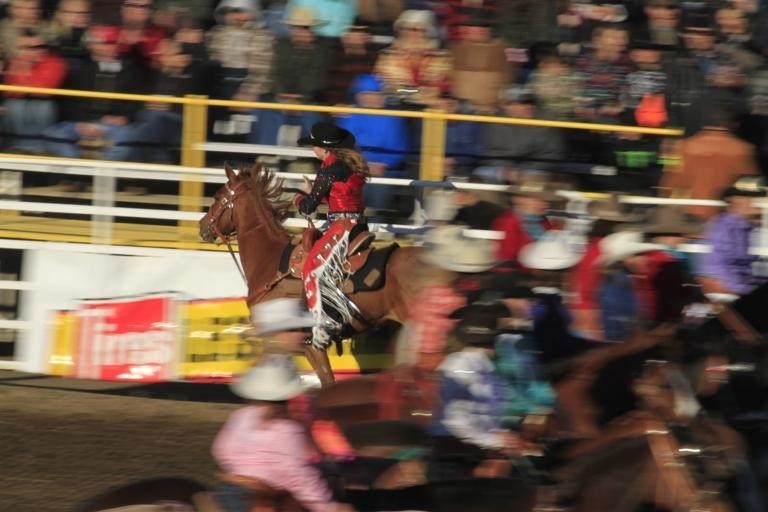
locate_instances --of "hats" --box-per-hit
[213,0,269,30]
[242,298,316,337]
[416,206,707,270]
[83,23,125,43]
[297,123,356,149]
[228,354,314,402]
[433,5,505,30]
[394,9,440,37]
[505,169,569,203]
[339,18,373,32]
[280,7,328,28]
[721,176,768,198]
[515,3,721,79]
[447,313,505,345]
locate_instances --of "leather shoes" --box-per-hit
[301,336,332,348]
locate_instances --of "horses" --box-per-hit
[197,159,451,387]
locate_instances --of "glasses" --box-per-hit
[15,44,49,50]
